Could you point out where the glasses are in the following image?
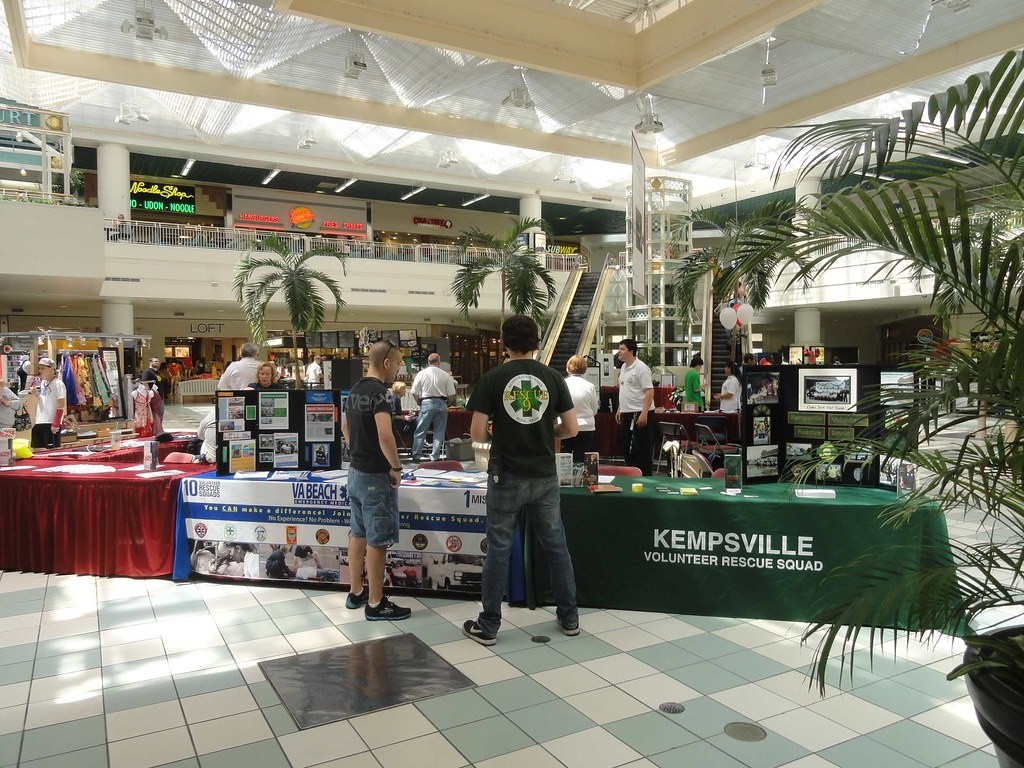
[382,338,396,369]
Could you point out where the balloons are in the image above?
[719,303,754,330]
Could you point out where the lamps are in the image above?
[552,164,576,184]
[297,127,317,149]
[761,38,776,86]
[436,145,458,169]
[115,101,149,124]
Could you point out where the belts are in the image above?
[420,396,446,402]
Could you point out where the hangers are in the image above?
[63,350,100,359]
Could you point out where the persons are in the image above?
[305,355,325,389]
[614,339,657,476]
[713,352,756,443]
[182,356,194,380]
[16,356,32,392]
[195,542,262,577]
[0,358,67,448]
[340,340,416,623]
[197,342,284,463]
[265,545,342,580]
[131,384,165,435]
[410,352,456,463]
[462,316,580,646]
[110,214,131,242]
[684,356,710,412]
[141,357,172,433]
[166,362,180,395]
[555,355,598,462]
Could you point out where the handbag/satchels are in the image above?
[12,404,32,431]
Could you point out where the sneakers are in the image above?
[365,594,411,621]
[556,617,581,636]
[462,619,497,645]
[345,585,369,609]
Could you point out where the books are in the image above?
[271,470,311,480]
[234,470,271,480]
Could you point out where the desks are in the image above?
[0,408,974,639]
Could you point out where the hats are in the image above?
[33,358,56,370]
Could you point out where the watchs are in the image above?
[393,463,403,472]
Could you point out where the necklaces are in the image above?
[43,375,55,387]
